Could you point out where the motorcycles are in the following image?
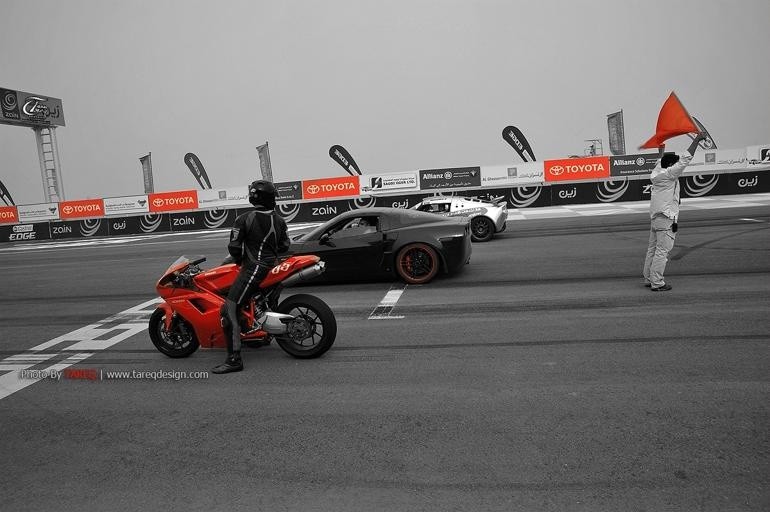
[147,251,338,360]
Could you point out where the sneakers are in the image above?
[211,358,244,374]
[651,283,672,291]
[645,283,651,287]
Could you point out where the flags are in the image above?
[639,134,666,150]
[655,92,699,145]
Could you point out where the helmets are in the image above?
[249,180,280,206]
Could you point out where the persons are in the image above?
[210,179,292,374]
[638,131,710,293]
[439,203,449,214]
[357,218,374,234]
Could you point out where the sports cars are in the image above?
[404,193,510,244]
[221,204,473,285]
